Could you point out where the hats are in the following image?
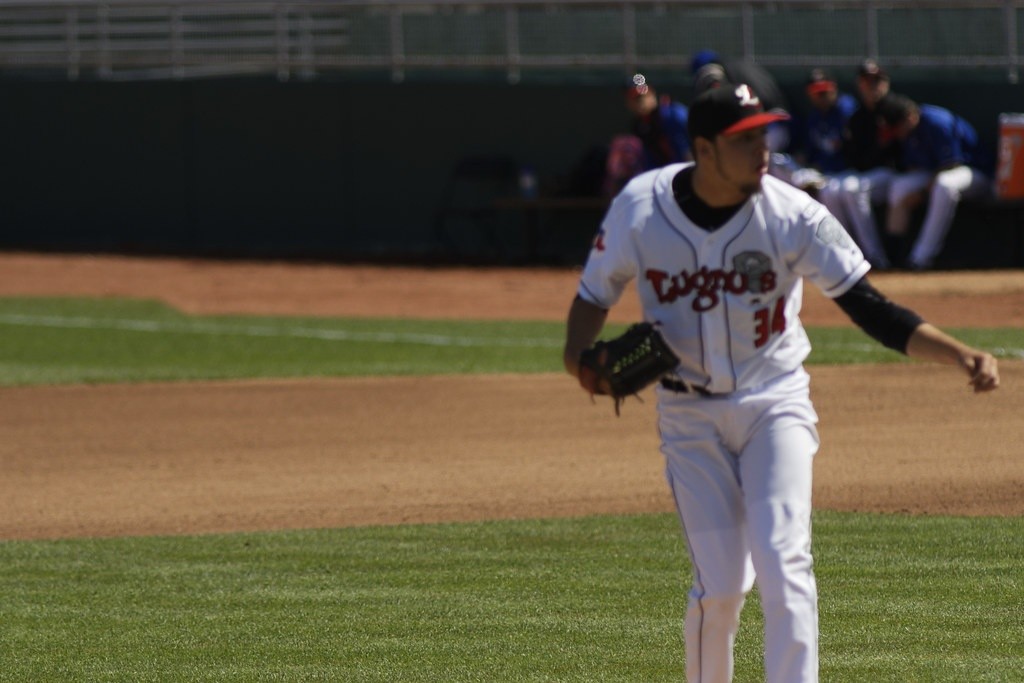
[687,84,791,140]
[622,70,657,104]
[852,57,891,80]
[690,52,727,91]
[806,68,837,95]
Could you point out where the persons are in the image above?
[562,82,998,683]
[606,52,985,268]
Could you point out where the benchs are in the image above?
[478,198,1024,264]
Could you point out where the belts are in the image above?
[658,378,711,398]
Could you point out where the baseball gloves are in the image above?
[578,321,681,399]
